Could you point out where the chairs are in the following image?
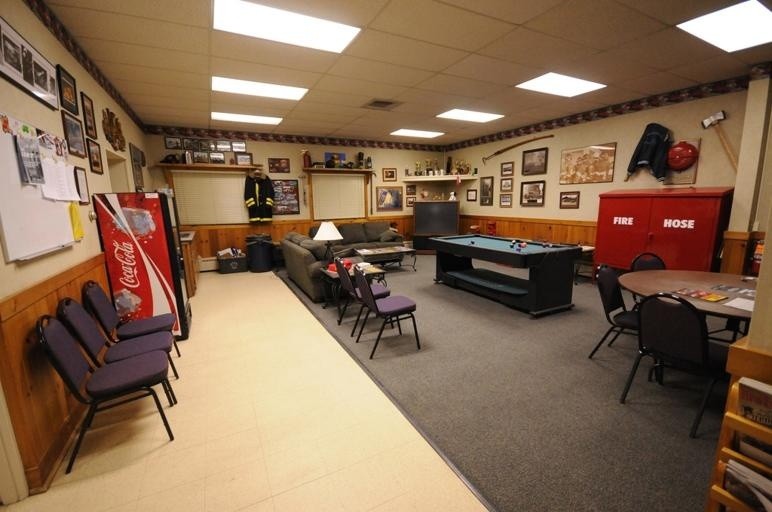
[36,313,174,474]
[588,263,641,360]
[620,293,730,441]
[334,259,393,337]
[56,298,179,404]
[353,265,421,359]
[82,280,181,377]
[630,253,666,312]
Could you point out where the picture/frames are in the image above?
[61,109,87,159]
[558,141,617,186]
[74,167,91,205]
[500,194,511,208]
[129,142,144,190]
[501,162,514,177]
[0,15,59,112]
[193,151,209,163]
[479,176,493,206]
[559,191,580,209]
[215,139,231,153]
[267,158,291,174]
[86,139,104,174]
[165,135,181,150]
[198,139,215,152]
[182,138,196,150]
[232,141,248,153]
[405,197,416,207]
[467,189,476,201]
[500,178,512,192]
[56,64,79,116]
[519,181,546,206]
[376,186,403,212]
[208,152,226,163]
[234,153,253,165]
[80,91,98,140]
[521,147,548,176]
[269,180,300,215]
[382,167,397,182]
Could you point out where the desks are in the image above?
[618,269,759,384]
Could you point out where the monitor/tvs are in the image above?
[412,201,459,236]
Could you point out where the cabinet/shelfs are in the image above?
[181,231,200,298]
[592,186,734,287]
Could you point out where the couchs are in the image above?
[310,222,404,250]
[280,232,372,303]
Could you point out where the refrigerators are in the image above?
[92,192,192,342]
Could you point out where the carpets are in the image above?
[272,254,772,512]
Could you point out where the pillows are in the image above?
[376,230,397,242]
[333,248,355,259]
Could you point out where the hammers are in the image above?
[700,111,738,173]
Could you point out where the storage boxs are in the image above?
[218,254,249,274]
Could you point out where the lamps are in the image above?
[313,221,343,270]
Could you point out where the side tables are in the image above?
[319,261,388,325]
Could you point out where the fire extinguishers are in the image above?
[750,238,764,274]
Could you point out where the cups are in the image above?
[429,171,433,176]
